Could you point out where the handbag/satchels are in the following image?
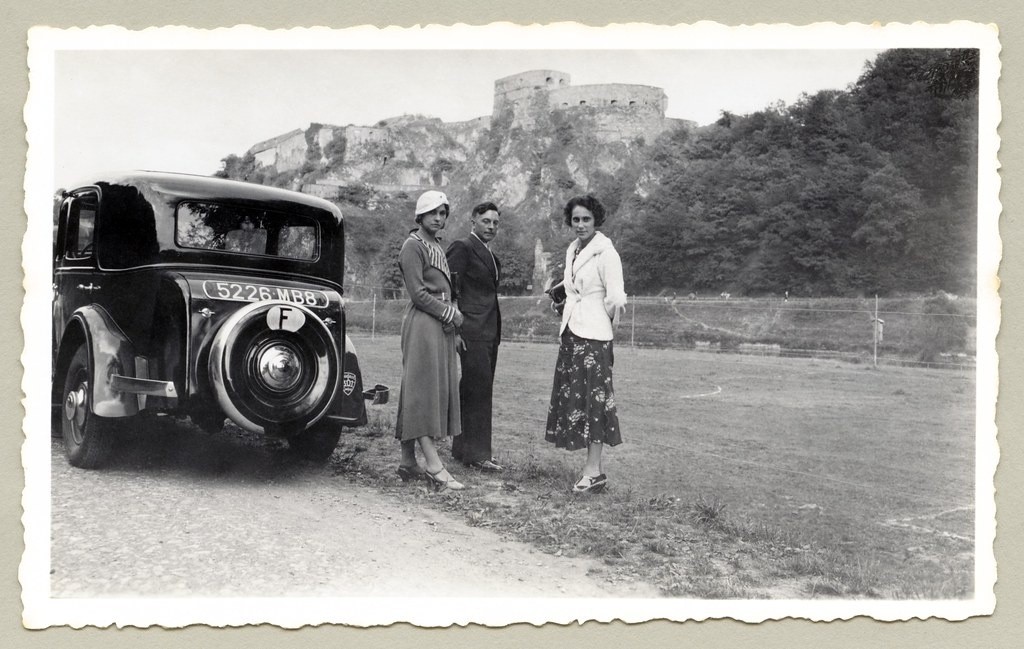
[543,279,566,315]
[450,271,462,302]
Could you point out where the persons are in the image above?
[446,202,503,473]
[545,197,625,494]
[395,190,464,489]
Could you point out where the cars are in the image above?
[53,170,390,469]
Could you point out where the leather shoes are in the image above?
[453,454,498,464]
[464,459,503,471]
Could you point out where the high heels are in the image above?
[426,466,464,490]
[572,472,608,496]
[398,463,427,482]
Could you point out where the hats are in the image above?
[414,189,450,215]
